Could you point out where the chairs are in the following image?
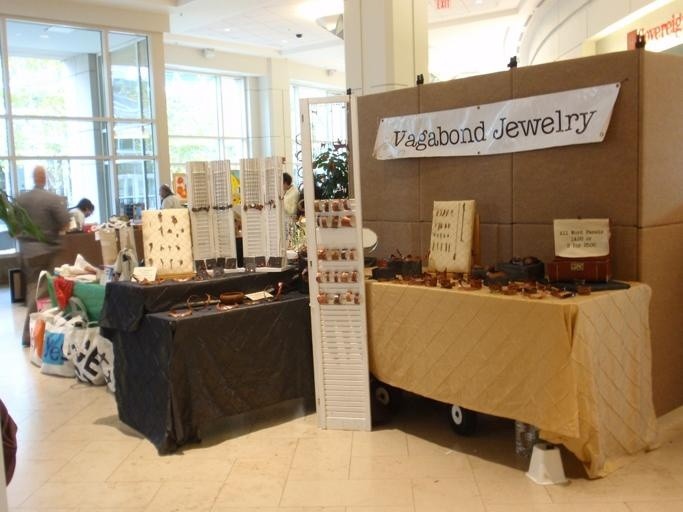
[61,230,144,267]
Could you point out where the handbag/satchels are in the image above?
[29,296,107,386]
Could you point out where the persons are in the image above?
[15,165,69,307]
[159,184,183,209]
[68,198,95,233]
[283,173,300,215]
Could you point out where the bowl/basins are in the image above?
[220,291,244,304]
